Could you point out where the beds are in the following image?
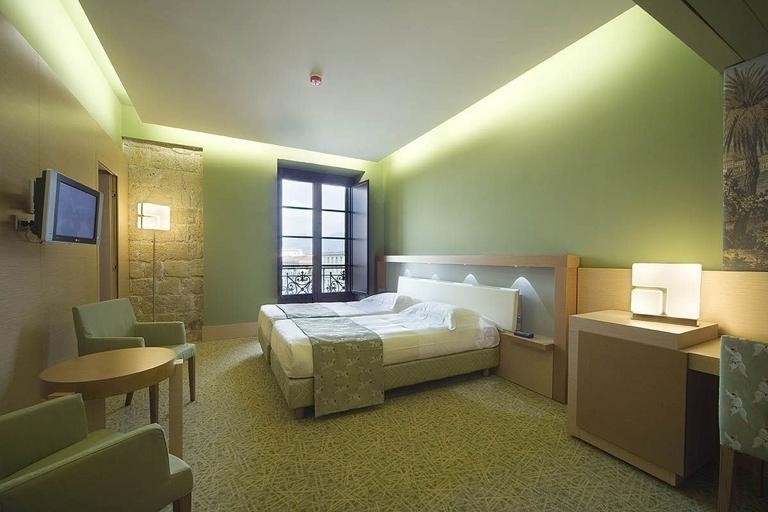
[267,315,502,420]
[255,301,419,370]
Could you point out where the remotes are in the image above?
[514,329,535,338]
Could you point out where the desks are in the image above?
[565,309,725,489]
[37,347,186,461]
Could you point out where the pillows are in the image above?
[363,291,419,310]
[406,299,481,330]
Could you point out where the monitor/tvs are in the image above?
[30,168,104,245]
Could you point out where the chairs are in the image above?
[0,388,194,512]
[710,331,768,512]
[69,295,197,425]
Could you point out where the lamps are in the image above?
[630,261,702,327]
[135,200,173,322]
